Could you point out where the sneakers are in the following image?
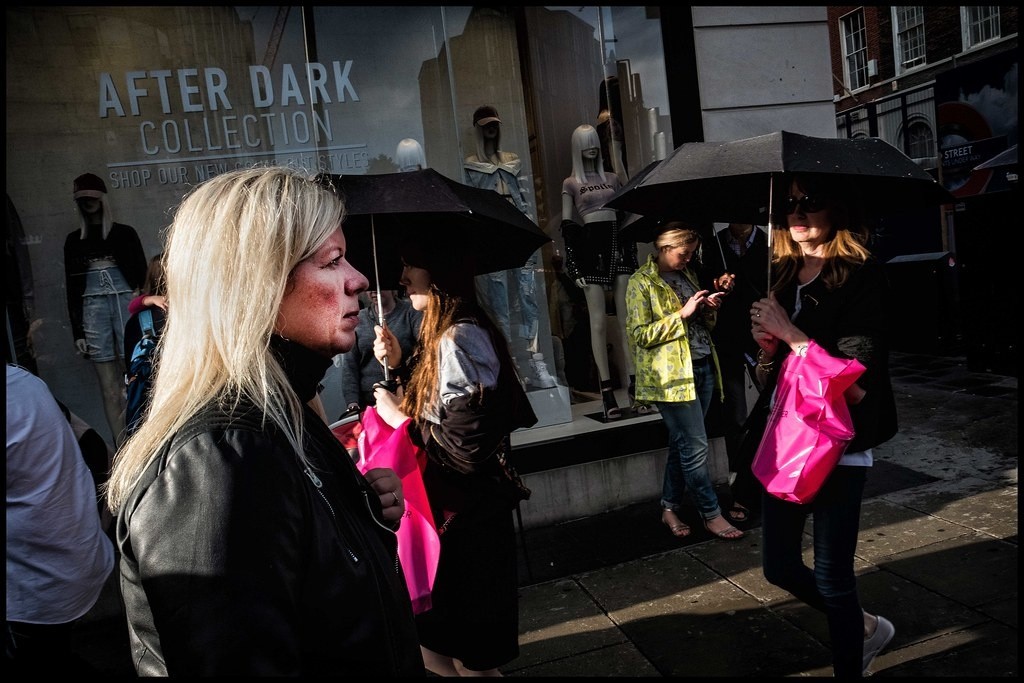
[861,616,895,675]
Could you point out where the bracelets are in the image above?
[759,363,773,372]
[756,348,775,366]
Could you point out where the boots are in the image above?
[511,357,526,393]
[529,353,558,389]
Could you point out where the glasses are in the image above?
[783,193,826,215]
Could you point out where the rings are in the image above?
[390,490,400,505]
[755,307,761,317]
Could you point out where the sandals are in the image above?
[728,497,750,522]
[703,512,746,540]
[662,507,692,538]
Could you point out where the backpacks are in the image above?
[125,312,160,437]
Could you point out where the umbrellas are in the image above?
[305,166,554,393]
[601,130,940,301]
[600,159,728,274]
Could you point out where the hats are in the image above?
[73,173,108,201]
[473,105,503,127]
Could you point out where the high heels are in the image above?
[602,386,622,419]
[627,381,652,414]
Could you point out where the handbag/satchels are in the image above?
[748,335,866,505]
[354,405,441,615]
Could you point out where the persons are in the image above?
[463,106,557,391]
[342,290,425,416]
[560,74,657,421]
[6,255,168,677]
[98,162,433,677]
[624,178,899,678]
[360,232,538,677]
[63,173,145,455]
[396,139,425,173]
